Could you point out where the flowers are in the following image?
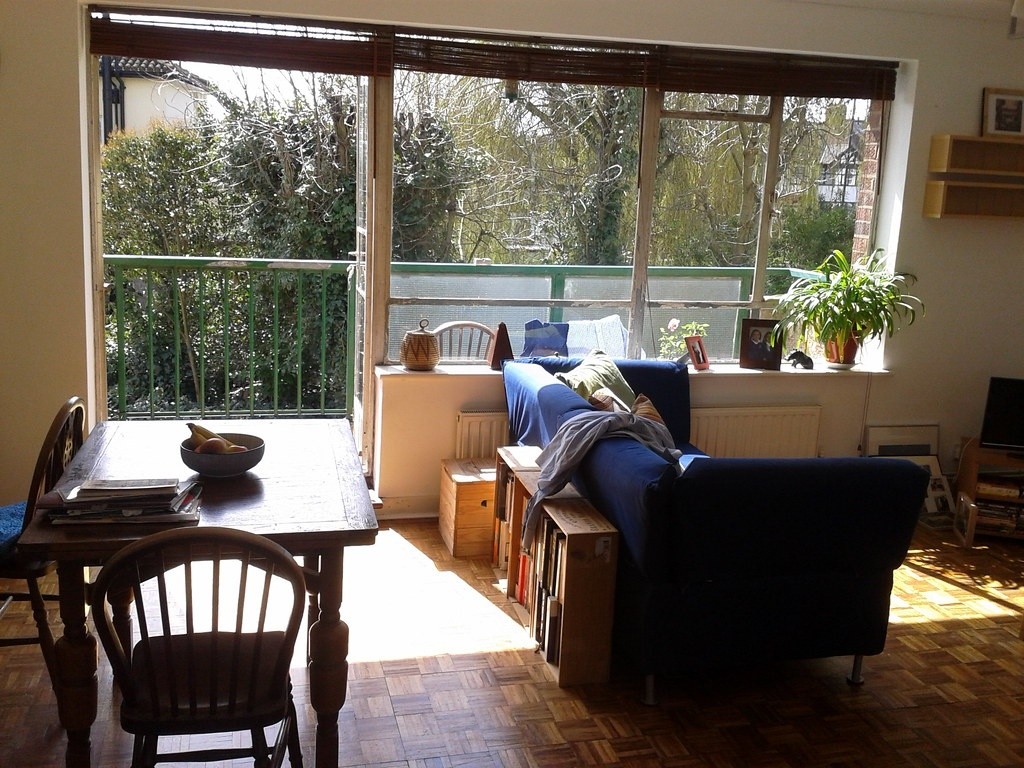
[658,318,710,363]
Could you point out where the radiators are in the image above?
[456,408,820,460]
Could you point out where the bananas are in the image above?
[187,423,235,447]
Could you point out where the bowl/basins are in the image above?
[179,433,266,476]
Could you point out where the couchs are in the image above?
[503,359,925,691]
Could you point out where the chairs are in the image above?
[91,525,307,767]
[1,399,91,726]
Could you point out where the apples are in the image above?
[195,438,249,452]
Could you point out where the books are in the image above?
[35,479,204,525]
[492,462,566,666]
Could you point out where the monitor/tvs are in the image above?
[979,376,1024,460]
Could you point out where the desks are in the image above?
[14,420,377,767]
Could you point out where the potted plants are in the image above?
[771,248,925,370]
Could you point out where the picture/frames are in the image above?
[683,336,710,370]
[741,319,784,370]
[982,87,1024,136]
[865,424,943,477]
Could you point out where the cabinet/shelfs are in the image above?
[492,444,619,685]
[439,458,496,557]
[925,133,1024,220]
[960,437,1023,539]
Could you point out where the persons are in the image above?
[692,345,701,364]
[749,329,775,358]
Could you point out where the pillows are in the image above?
[555,350,636,411]
